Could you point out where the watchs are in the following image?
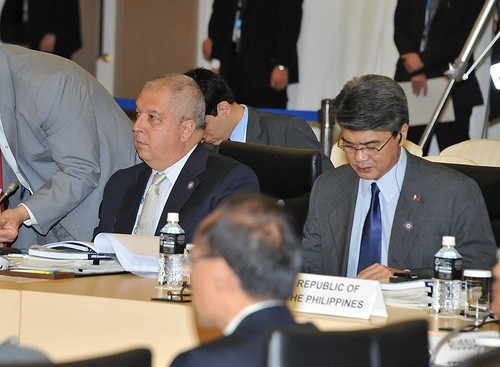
[278,64,286,71]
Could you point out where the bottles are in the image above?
[157,212,185,290]
[429,236,461,313]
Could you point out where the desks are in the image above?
[0,245,484,367]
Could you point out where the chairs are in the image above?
[217,138,500,278]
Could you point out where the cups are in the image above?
[461,269,494,319]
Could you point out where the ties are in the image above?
[429,0,439,22]
[356,183,382,275]
[135,172,167,237]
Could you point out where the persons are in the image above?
[171,194,323,367]
[202,0,303,110]
[450,262,500,367]
[0,41,142,251]
[296,74,495,283]
[0,0,84,61]
[394,0,486,157]
[189,68,336,177]
[90,74,261,258]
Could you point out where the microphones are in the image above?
[0,179,20,204]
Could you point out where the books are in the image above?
[382,279,466,309]
[2,233,162,273]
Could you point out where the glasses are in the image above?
[336,130,396,157]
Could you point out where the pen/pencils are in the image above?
[10,270,56,274]
